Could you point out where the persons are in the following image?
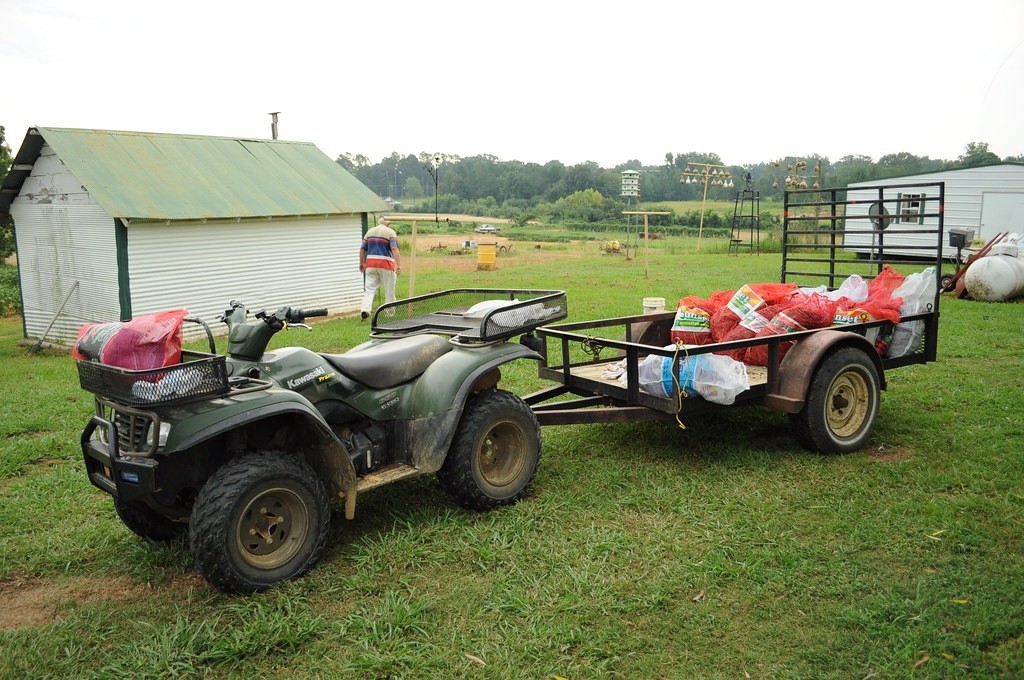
[358,216,403,321]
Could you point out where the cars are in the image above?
[475,224,497,233]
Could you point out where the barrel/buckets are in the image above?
[478,244,496,271]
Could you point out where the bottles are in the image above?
[132,380,162,400]
[158,366,206,395]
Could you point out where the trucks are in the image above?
[74,182,946,595]
